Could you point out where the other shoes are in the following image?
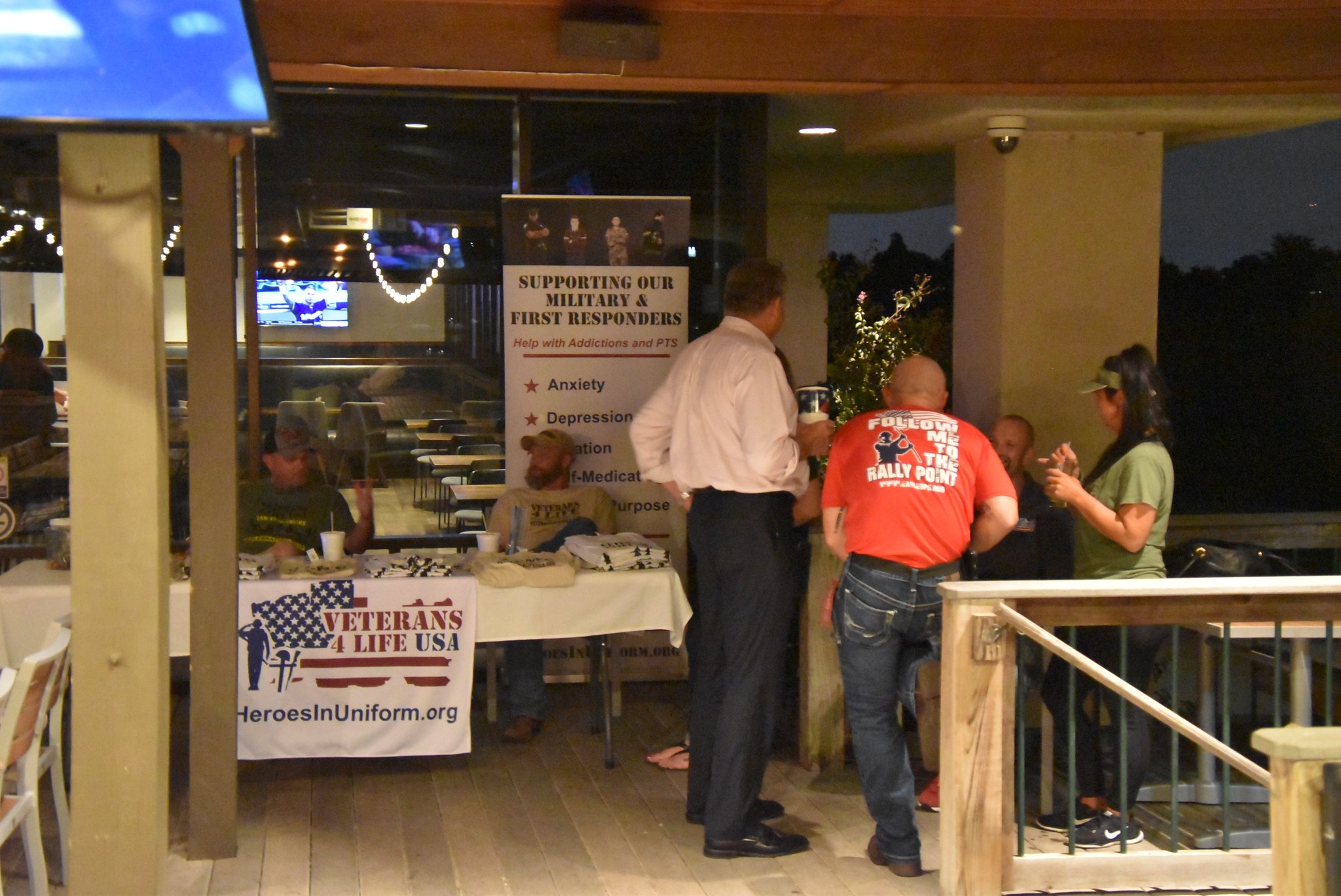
[648,740,690,771]
[868,834,922,877]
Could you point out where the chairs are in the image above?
[333,402,390,489]
[411,401,505,554]
[274,401,330,483]
[0,626,72,896]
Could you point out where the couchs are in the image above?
[47,364,457,477]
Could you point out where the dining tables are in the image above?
[48,401,68,443]
[0,553,674,771]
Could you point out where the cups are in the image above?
[796,386,829,424]
[1048,459,1074,508]
[320,531,346,562]
[475,532,502,553]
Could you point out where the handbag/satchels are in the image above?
[1173,537,1307,575]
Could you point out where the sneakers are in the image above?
[918,772,940,812]
[1064,809,1144,847]
[1037,808,1100,831]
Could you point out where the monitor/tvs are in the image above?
[256,274,348,326]
[0,0,278,139]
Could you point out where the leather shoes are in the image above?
[703,826,809,858]
[499,716,539,743]
[686,799,784,823]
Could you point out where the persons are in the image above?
[606,216,630,266]
[916,415,1079,812]
[486,429,617,741]
[561,215,587,266]
[239,416,375,567]
[0,328,58,450]
[628,258,836,859]
[520,208,551,265]
[1037,344,1174,848]
[640,210,667,266]
[820,356,1019,878]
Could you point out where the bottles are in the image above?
[43,518,72,571]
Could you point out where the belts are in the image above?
[850,552,961,579]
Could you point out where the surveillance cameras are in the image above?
[986,114,1028,154]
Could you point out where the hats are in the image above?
[1076,368,1157,397]
[521,429,575,464]
[0,328,43,357]
[261,430,318,460]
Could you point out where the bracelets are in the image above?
[674,491,694,509]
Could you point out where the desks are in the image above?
[429,454,506,531]
[416,433,505,507]
[1137,620,1341,849]
[404,419,505,477]
[261,408,341,414]
[450,485,505,553]
